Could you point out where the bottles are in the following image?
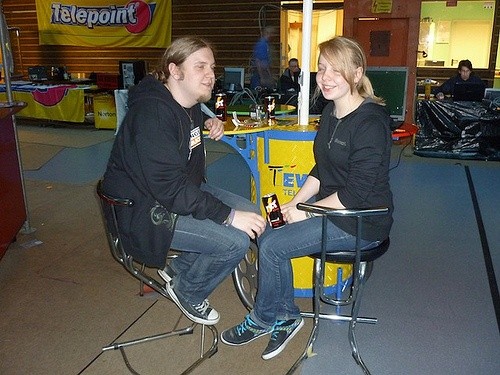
[425,80,430,100]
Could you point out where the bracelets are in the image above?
[222,208,236,228]
[305,210,315,218]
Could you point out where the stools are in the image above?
[96,178,219,375]
[283,202,391,375]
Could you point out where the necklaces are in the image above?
[328,94,360,140]
[165,80,193,124]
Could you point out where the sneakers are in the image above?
[262,317,304,360]
[165,279,221,325]
[220,314,275,346]
[158,262,210,306]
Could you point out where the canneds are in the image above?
[214,93,227,123]
[264,96,276,118]
[262,193,286,230]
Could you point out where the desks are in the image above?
[209,97,297,115]
[202,116,278,203]
[0,81,99,127]
[388,122,419,171]
[414,98,500,161]
[92,93,117,130]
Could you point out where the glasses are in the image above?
[290,65,299,68]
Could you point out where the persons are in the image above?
[276,58,301,115]
[249,24,273,93]
[434,60,489,102]
[98,36,268,326]
[220,36,395,361]
